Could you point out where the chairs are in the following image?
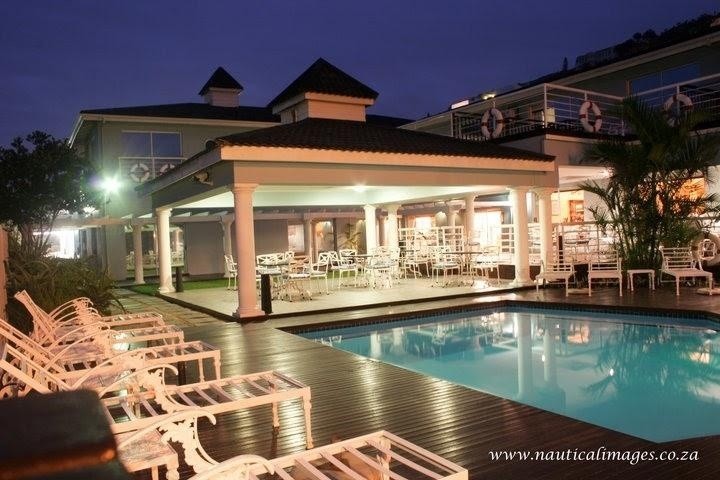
[0,282,469,480]
[124,244,713,303]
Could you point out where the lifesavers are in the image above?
[692,231,720,267]
[662,94,693,128]
[579,100,602,132]
[481,108,504,140]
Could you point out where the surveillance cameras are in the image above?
[193,171,209,184]
